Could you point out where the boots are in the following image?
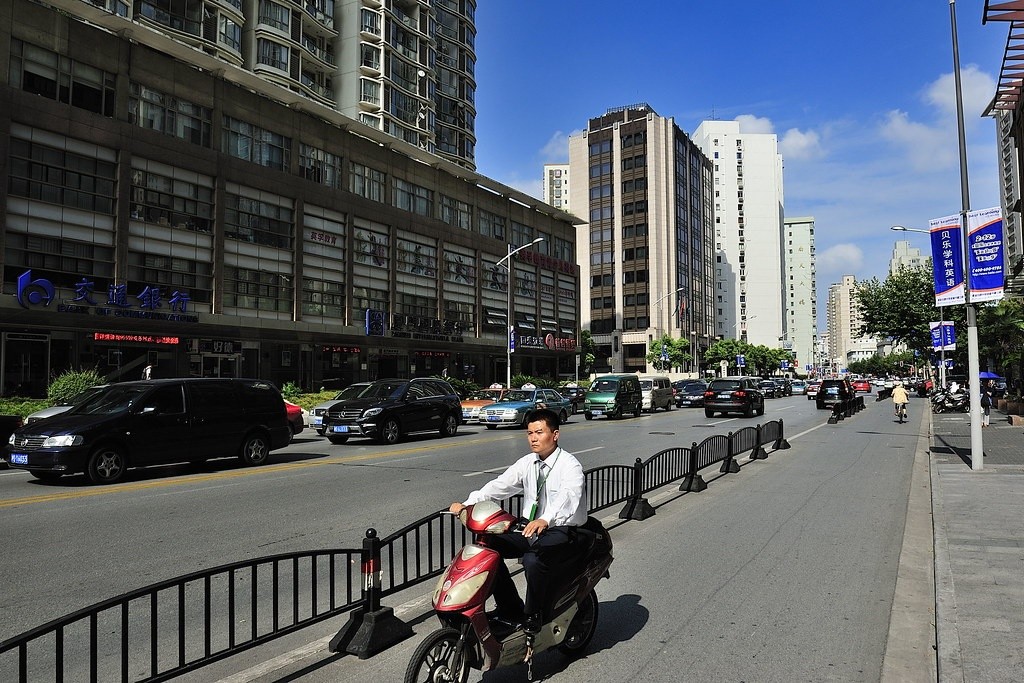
[903,409,907,419]
[895,409,898,416]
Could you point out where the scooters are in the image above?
[930,382,971,414]
[403,499,614,683]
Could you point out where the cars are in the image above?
[852,380,872,394]
[847,373,923,389]
[995,376,1006,392]
[671,378,711,409]
[750,376,822,401]
[946,375,968,384]
[283,401,305,441]
[307,380,377,437]
[559,382,590,414]
[460,382,517,426]
[478,383,573,430]
[22,384,110,428]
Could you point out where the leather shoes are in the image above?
[486,608,510,622]
[522,612,541,633]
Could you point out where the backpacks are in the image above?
[141,367,151,380]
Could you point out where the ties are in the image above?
[527,462,547,547]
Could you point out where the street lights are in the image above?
[495,237,544,391]
[732,314,758,376]
[778,327,800,379]
[652,287,685,373]
[890,225,947,391]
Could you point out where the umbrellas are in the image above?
[979,372,999,378]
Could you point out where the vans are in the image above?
[583,373,643,420]
[638,376,675,413]
[3,377,292,485]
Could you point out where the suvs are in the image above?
[703,376,764,418]
[321,376,464,444]
[816,379,854,409]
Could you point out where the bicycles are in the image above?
[896,402,906,424]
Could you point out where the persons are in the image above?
[891,382,909,419]
[955,385,965,394]
[920,380,926,398]
[142,363,154,380]
[449,410,587,637]
[980,380,992,428]
